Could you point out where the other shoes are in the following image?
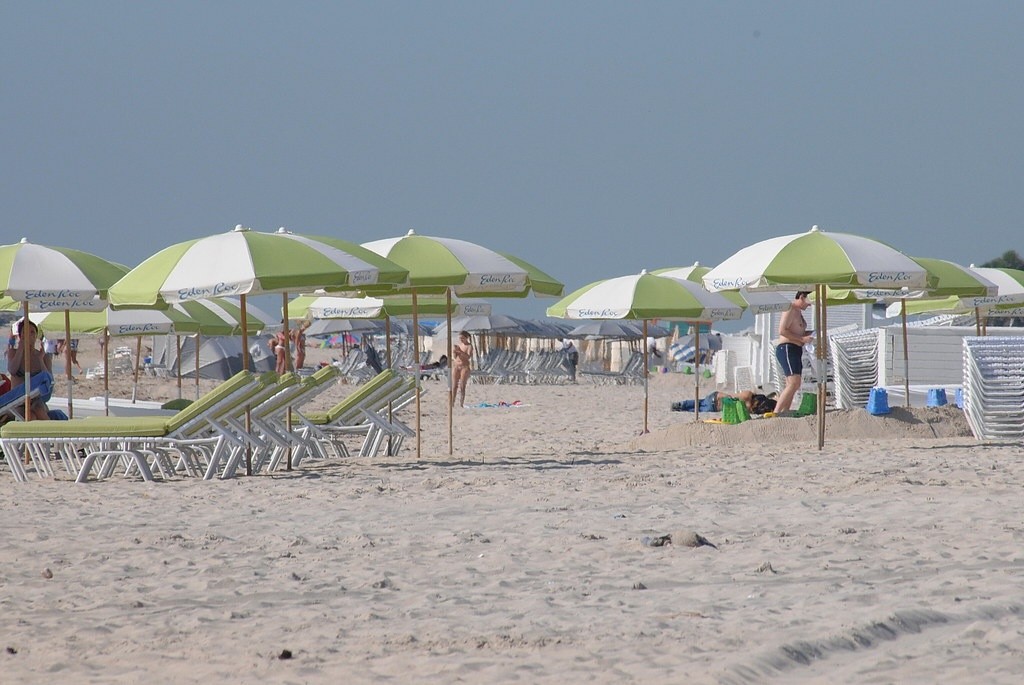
[671,401,679,412]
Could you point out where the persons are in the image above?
[399,355,447,371]
[772,292,817,418]
[318,344,359,369]
[449,330,473,410]
[555,335,579,385]
[673,391,775,414]
[7,319,53,421]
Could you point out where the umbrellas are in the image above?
[107,224,411,476]
[702,224,940,450]
[0,221,1023,476]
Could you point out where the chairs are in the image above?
[713,301,1024,440]
[467,347,646,386]
[0,347,450,483]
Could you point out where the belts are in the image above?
[711,391,717,412]
